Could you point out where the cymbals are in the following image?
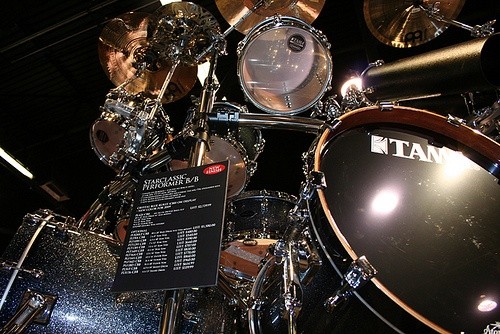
[95,11,199,105]
[363,0,465,49]
[216,0,326,42]
[147,2,221,67]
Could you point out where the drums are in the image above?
[246,99,500,334]
[116,179,138,245]
[236,12,335,116]
[89,89,170,174]
[164,96,266,202]
[217,189,300,283]
